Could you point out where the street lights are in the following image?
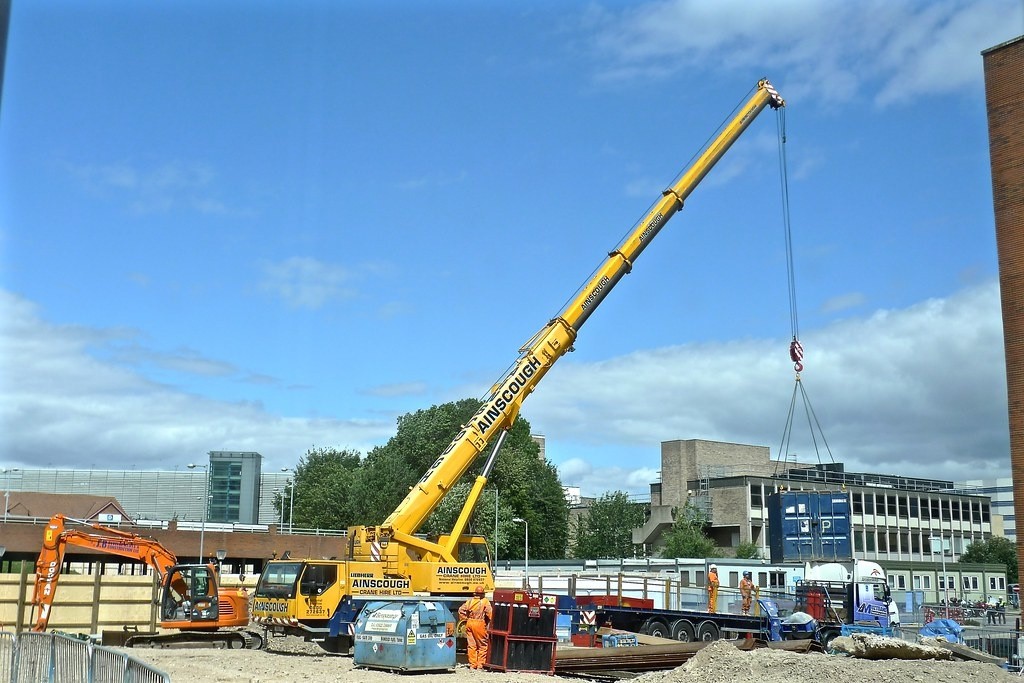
[2,469,20,515]
[186,463,208,565]
[928,537,950,620]
[513,517,529,591]
[281,467,294,535]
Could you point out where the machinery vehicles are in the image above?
[252,79,784,652]
[29,514,262,650]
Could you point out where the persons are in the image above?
[987,596,1006,625]
[708,564,719,614]
[458,585,493,672]
[740,570,756,616]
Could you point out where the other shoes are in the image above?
[469,668,487,673]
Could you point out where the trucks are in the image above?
[556,559,902,655]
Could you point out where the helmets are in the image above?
[998,596,1003,599]
[710,563,717,569]
[743,570,750,576]
[474,586,488,593]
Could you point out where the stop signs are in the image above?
[1013,585,1021,593]
